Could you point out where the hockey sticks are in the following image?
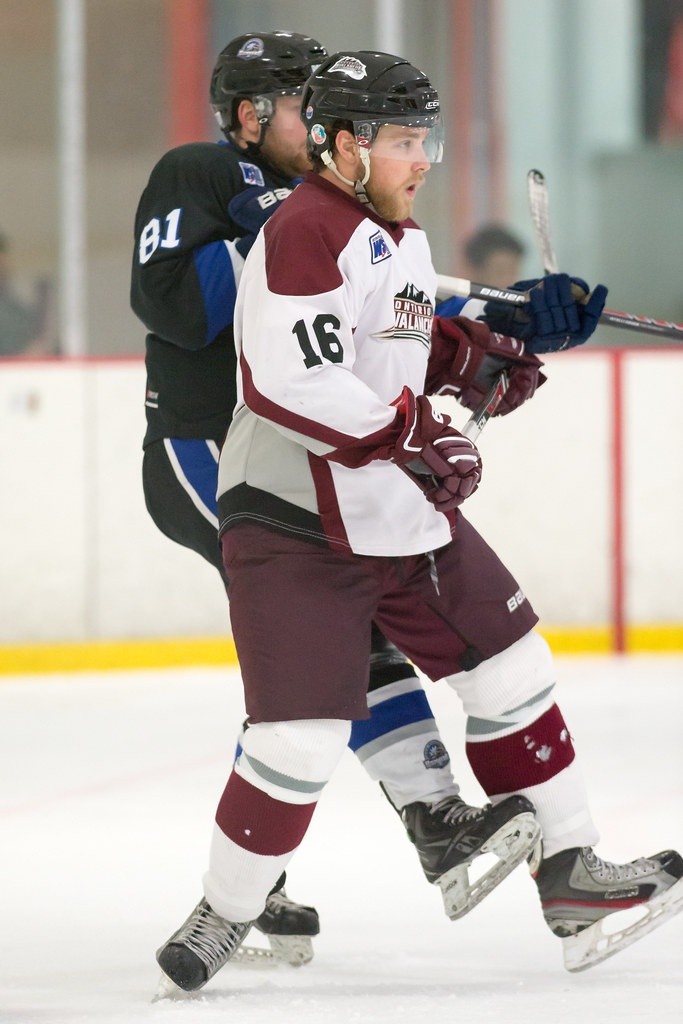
[434,273,683,344]
[423,168,560,490]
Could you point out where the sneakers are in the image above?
[229,869,320,971]
[526,839,683,973]
[378,780,542,921]
[150,896,255,1005]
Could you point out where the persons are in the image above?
[463,226,527,289]
[130,31,610,969]
[1,231,59,357]
[149,50,683,1002]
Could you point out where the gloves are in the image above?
[228,186,294,255]
[388,385,482,512]
[475,273,608,354]
[423,316,547,417]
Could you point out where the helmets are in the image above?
[209,30,329,134]
[299,51,446,165]
